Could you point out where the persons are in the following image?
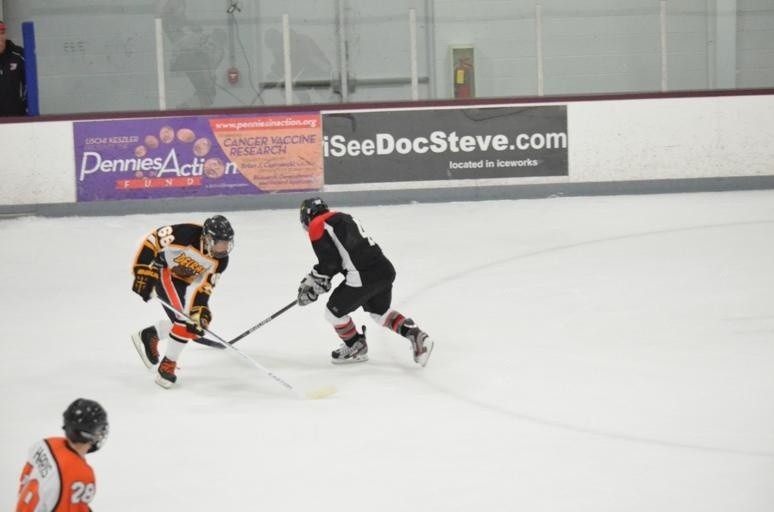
[0,21,27,117]
[132,215,233,388]
[297,199,431,365]
[15,399,107,511]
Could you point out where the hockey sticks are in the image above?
[192,300,298,349]
[150,292,337,398]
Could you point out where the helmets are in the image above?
[62,396,110,452]
[199,214,236,260]
[300,196,330,231]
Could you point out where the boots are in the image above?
[157,355,178,384]
[402,316,431,364]
[139,325,161,365]
[330,324,369,359]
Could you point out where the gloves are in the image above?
[296,269,332,307]
[131,275,157,303]
[186,290,212,336]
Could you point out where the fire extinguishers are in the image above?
[454,57,473,100]
